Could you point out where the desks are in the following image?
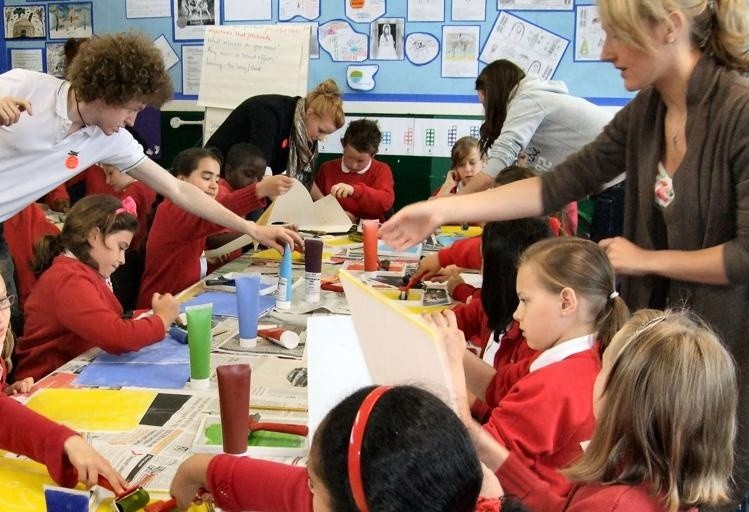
[1,223,483,511]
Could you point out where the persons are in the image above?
[1,1,749,512]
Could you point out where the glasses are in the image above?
[0,293,17,310]
